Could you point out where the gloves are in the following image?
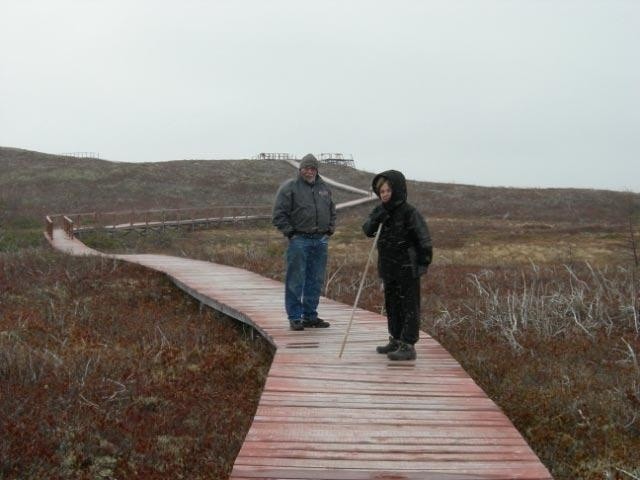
[374,208,391,224]
[417,248,433,267]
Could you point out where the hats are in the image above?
[299,154,319,173]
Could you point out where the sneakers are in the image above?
[377,335,402,354]
[290,319,304,331]
[302,317,330,328]
[388,341,417,360]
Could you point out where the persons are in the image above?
[271,153,338,331]
[361,171,433,361]
